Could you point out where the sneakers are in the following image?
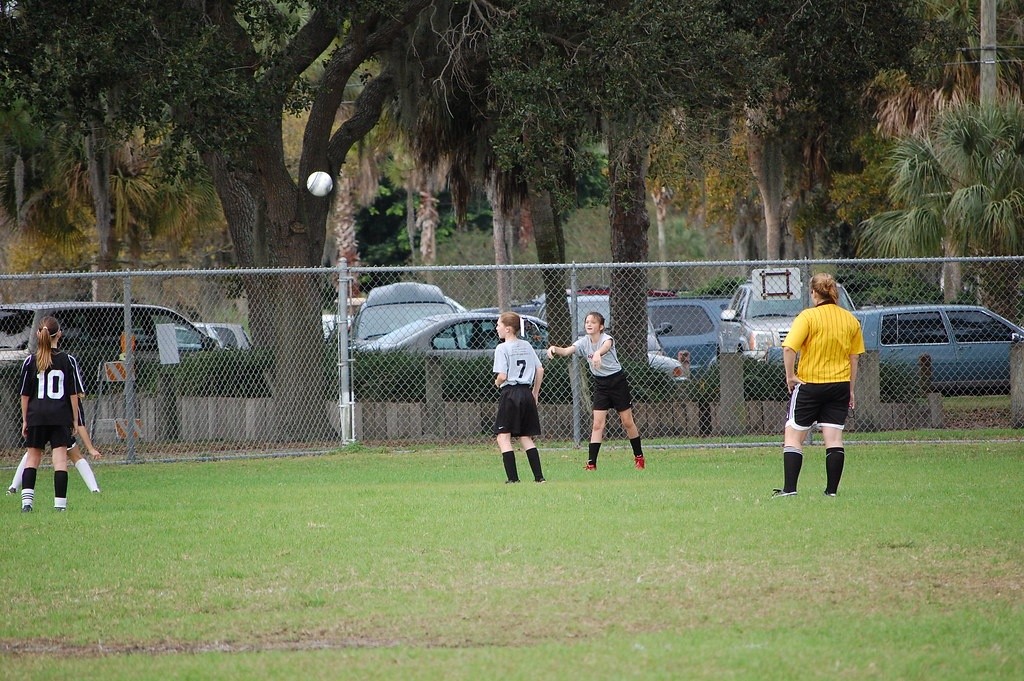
[582,460,596,471]
[634,455,645,470]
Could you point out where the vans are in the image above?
[352,283,469,346]
[0,302,220,360]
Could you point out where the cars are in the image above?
[358,313,585,360]
[322,297,365,340]
[192,323,252,350]
[851,305,1024,402]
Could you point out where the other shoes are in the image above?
[822,491,836,498]
[505,479,520,484]
[6,487,16,495]
[21,505,31,513]
[533,479,546,483]
[54,506,66,513]
[770,488,798,499]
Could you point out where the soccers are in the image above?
[306,170,334,198]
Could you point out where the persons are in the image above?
[769,273,865,499]
[548,312,645,470]
[493,310,546,484]
[6,317,103,511]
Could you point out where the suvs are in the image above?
[721,278,859,367]
[474,285,731,366]
